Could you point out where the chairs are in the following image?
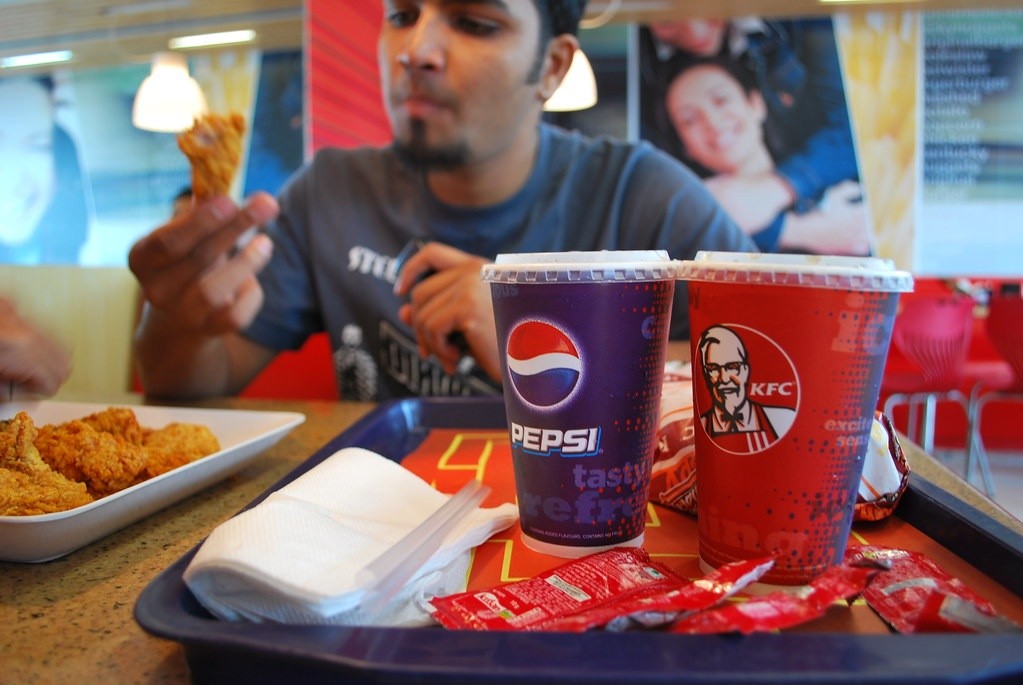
[888,291,1023,499]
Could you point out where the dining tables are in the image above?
[0,401,1023,685]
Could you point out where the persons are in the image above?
[638,17,872,257]
[126,186,193,397]
[126,1,762,404]
[0,75,98,267]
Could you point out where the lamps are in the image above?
[542,48,599,112]
[132,51,210,133]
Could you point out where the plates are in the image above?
[0,398,306,563]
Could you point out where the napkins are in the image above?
[181,447,520,625]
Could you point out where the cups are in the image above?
[677,250,914,596]
[482,249,672,559]
